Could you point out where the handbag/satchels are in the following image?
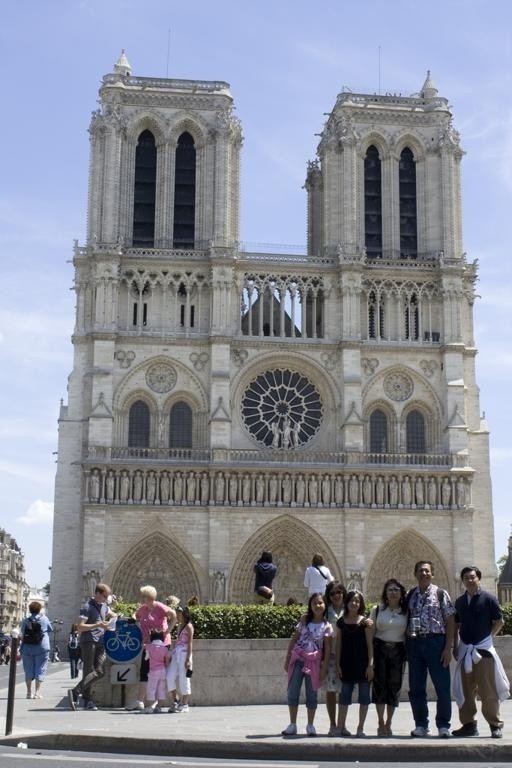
[255,584,274,600]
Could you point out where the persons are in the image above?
[281,554,374,737]
[0,584,198,715]
[452,566,510,738]
[371,577,409,737]
[406,561,458,737]
[253,550,277,606]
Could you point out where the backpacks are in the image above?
[68,633,80,650]
[23,617,45,645]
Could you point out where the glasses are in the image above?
[329,590,344,596]
[387,587,401,593]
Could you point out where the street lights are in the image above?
[52,615,66,663]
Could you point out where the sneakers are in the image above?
[451,721,479,737]
[411,726,429,738]
[438,727,450,738]
[281,724,297,735]
[377,727,392,737]
[490,722,503,738]
[327,724,351,737]
[357,728,367,738]
[305,725,317,736]
[26,693,44,700]
[67,689,189,714]
